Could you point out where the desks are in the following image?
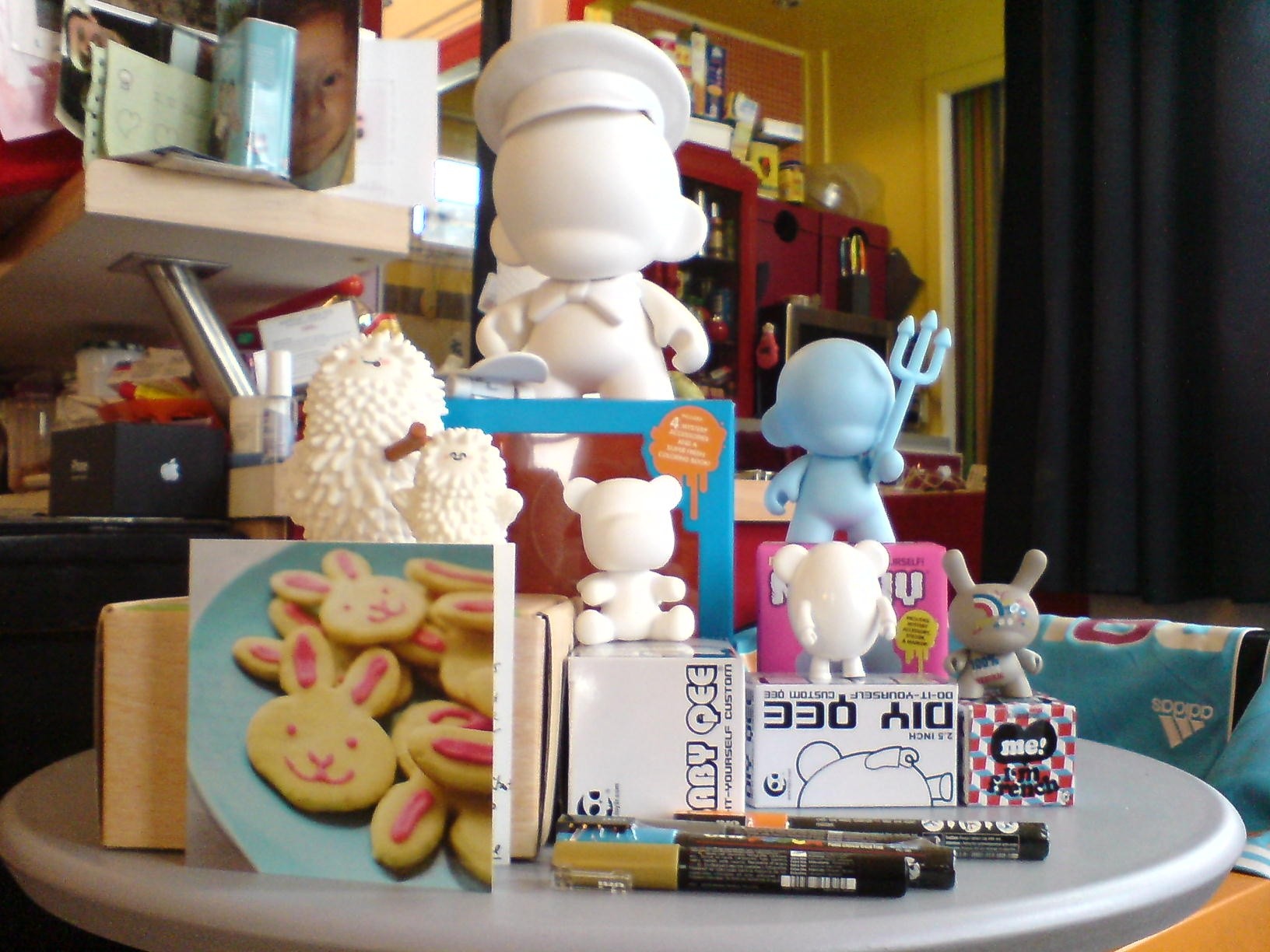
[0,715,1242,952]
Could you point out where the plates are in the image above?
[0,741,1247,952]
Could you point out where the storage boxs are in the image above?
[50,399,1079,896]
[214,19,298,176]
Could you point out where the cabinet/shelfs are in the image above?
[0,0,405,626]
[646,140,893,435]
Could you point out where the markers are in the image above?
[552,840,909,899]
[672,810,1050,863]
[554,814,957,891]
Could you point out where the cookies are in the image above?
[231,548,493,887]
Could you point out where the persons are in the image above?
[266,0,360,193]
[64,8,171,71]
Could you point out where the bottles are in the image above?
[705,294,729,341]
[708,202,723,257]
